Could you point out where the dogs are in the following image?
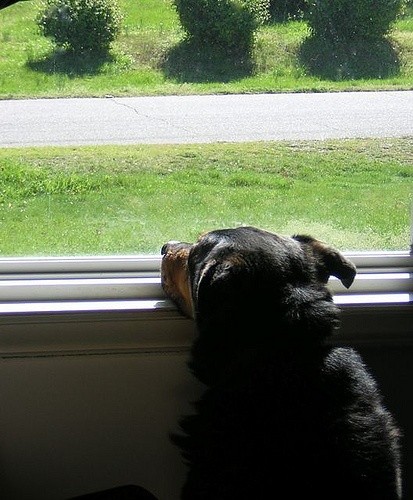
[159,224,403,500]
